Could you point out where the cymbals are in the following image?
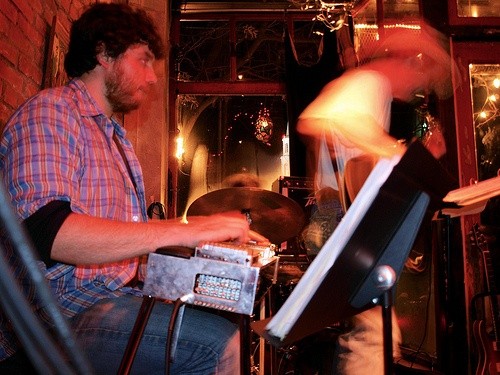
[187,188,306,241]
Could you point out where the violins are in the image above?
[469,224,500,375]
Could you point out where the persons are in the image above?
[0,1,262,374]
